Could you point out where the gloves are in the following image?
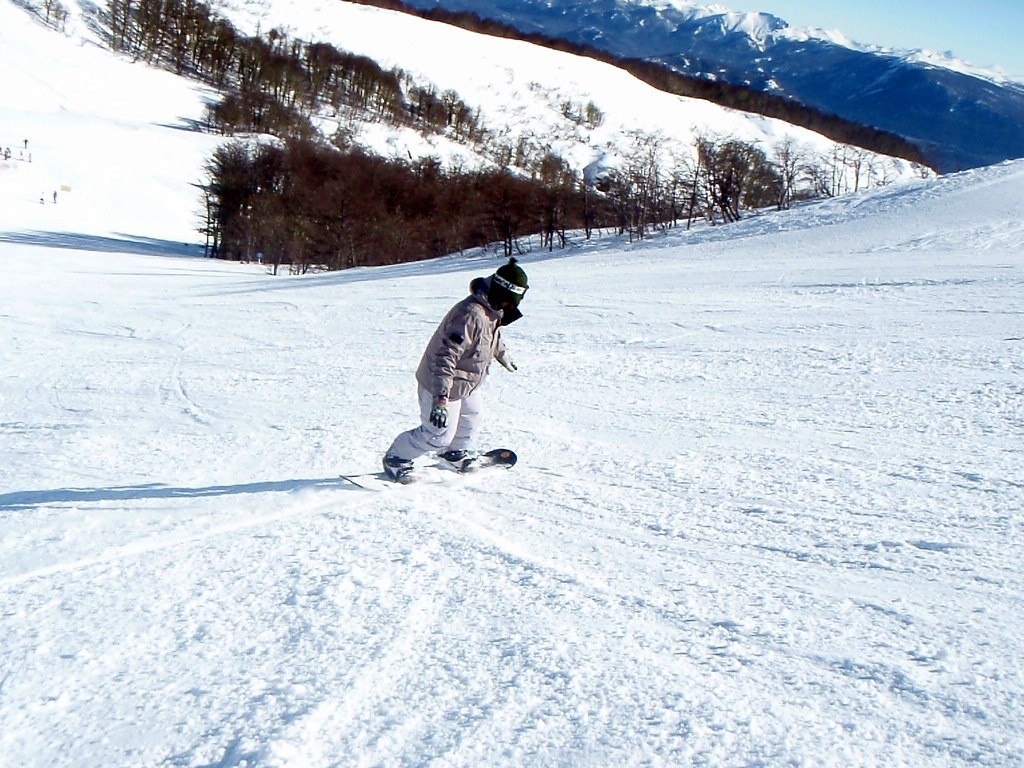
[495,350,518,372]
[429,394,449,429]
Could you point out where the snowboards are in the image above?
[339,448,518,491]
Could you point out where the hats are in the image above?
[488,258,529,307]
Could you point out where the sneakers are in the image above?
[436,448,481,474]
[382,455,413,485]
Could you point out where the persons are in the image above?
[40,191,57,204]
[0,139,31,161]
[382,258,528,484]
